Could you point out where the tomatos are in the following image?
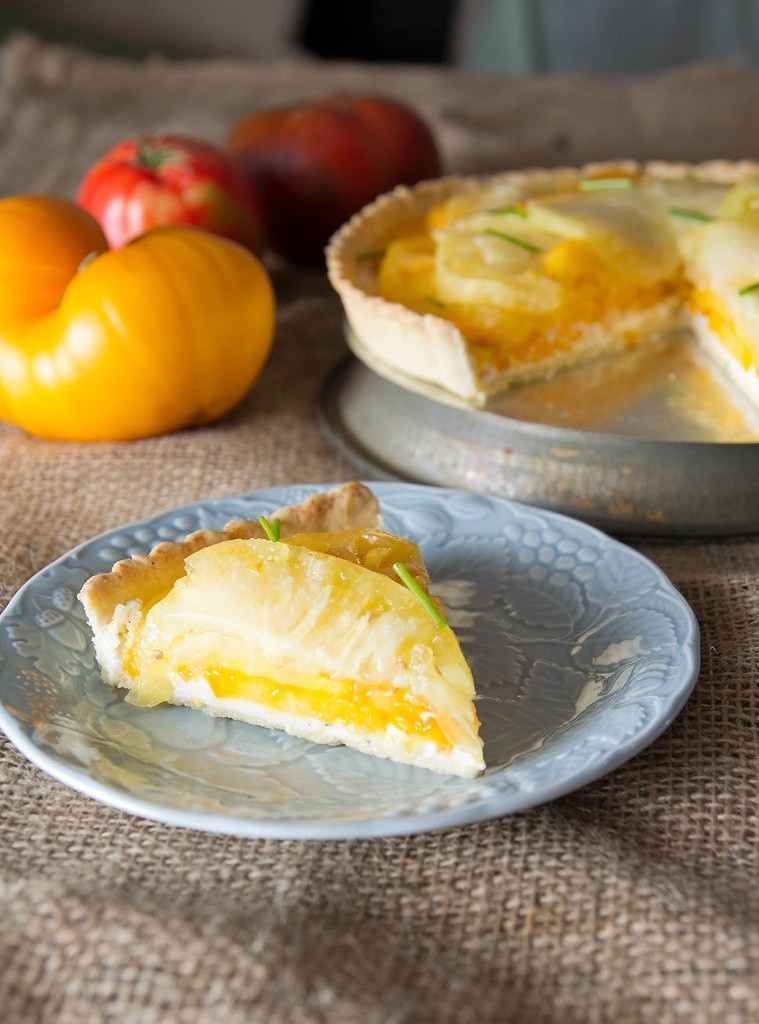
[1,92,442,440]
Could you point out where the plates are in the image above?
[0,479,702,842]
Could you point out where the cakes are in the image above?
[325,156,759,439]
[81,482,485,782]
